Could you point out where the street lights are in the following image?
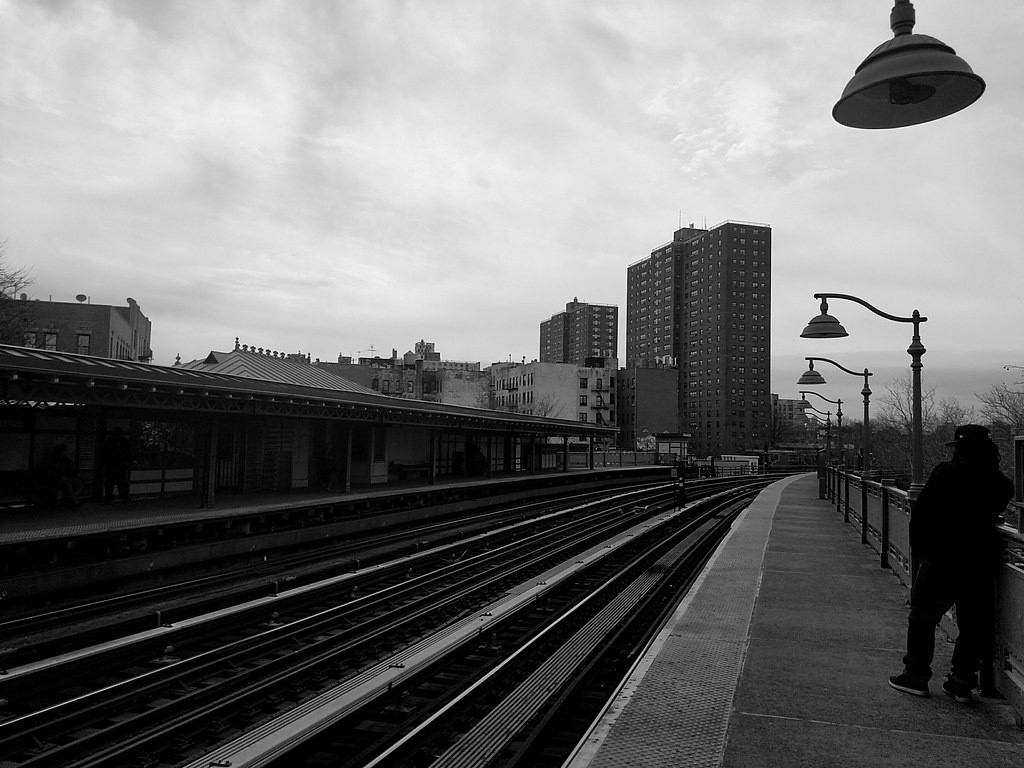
[800,294,927,499]
[795,404,832,464]
[797,357,874,479]
[794,391,843,470]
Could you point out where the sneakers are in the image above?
[942,680,975,703]
[889,672,929,696]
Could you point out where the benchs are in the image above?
[0,469,44,514]
[390,460,432,482]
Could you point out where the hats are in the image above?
[943,424,993,445]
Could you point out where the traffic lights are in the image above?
[678,476,684,488]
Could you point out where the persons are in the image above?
[99,427,130,504]
[889,425,1015,704]
